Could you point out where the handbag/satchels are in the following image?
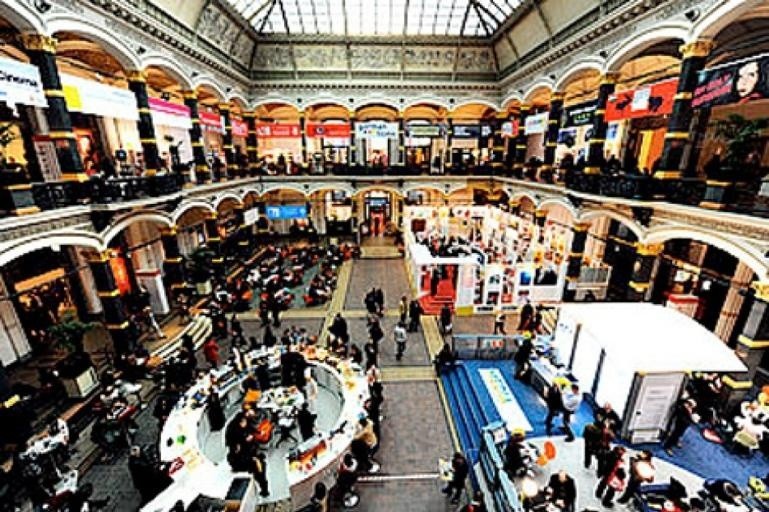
[608,473,625,492]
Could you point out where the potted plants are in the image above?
[41,317,102,400]
[187,245,220,296]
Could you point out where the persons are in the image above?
[726,59,768,103]
[0,134,768,512]
